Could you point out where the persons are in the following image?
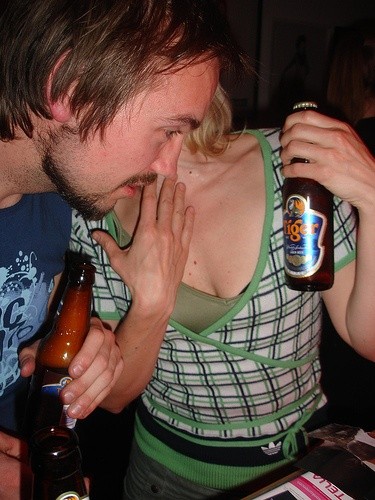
[0,0,220,500]
[61,81,375,500]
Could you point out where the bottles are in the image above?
[18,260,96,500]
[281,102,334,291]
